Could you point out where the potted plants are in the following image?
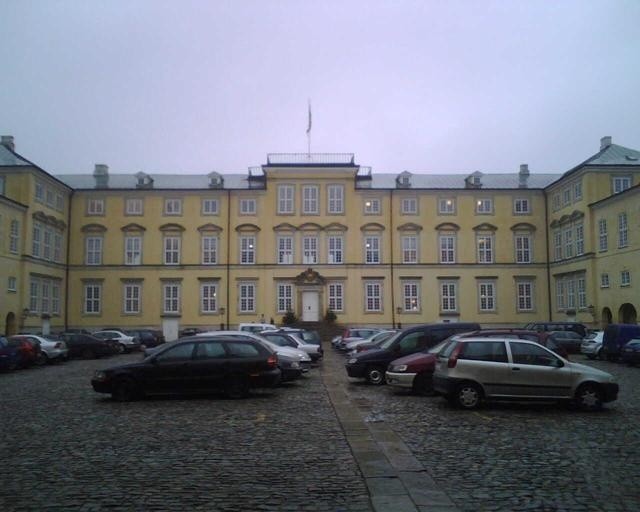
[325,311,337,323]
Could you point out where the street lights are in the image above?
[218,306,226,330]
[395,306,404,330]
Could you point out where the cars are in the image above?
[0,335,42,374]
[197,322,324,383]
[331,329,394,359]
[433,338,620,412]
[622,339,640,368]
[524,320,592,355]
[9,334,68,367]
[90,334,284,401]
[178,327,203,339]
[39,327,164,361]
[385,329,571,397]
[580,331,608,361]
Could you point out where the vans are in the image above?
[602,323,640,364]
[345,321,481,386]
